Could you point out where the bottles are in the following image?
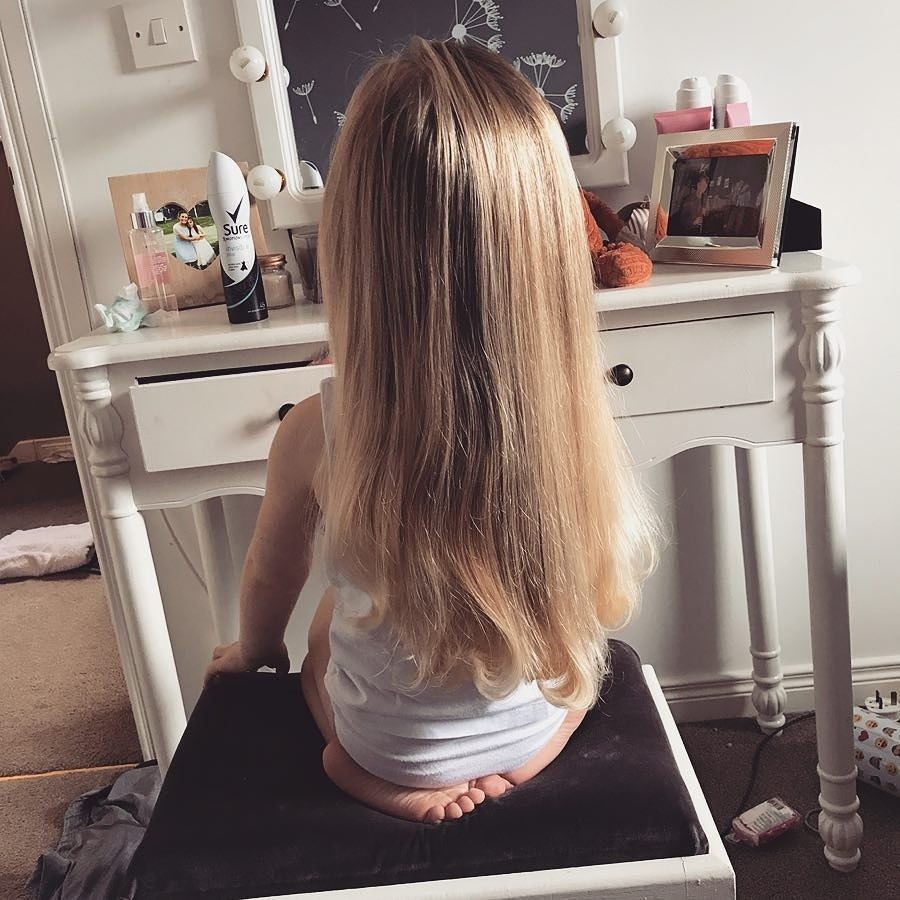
[257,254,295,310]
[207,151,268,325]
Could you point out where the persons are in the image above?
[172,210,217,269]
[676,173,710,236]
[203,38,664,825]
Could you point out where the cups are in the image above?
[292,230,323,304]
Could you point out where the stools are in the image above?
[129,638,737,900]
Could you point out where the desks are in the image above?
[46,248,864,873]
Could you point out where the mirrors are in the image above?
[232,0,630,231]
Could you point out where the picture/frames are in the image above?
[644,122,800,270]
[110,162,269,313]
